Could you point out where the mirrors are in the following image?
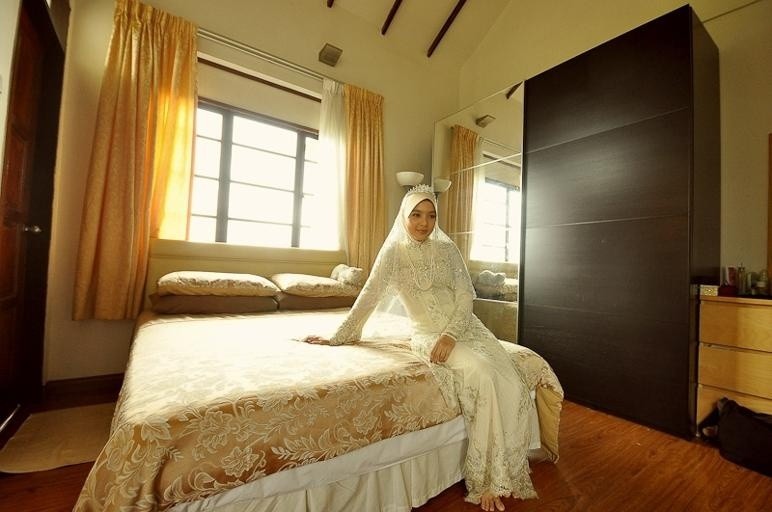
[431,82,522,303]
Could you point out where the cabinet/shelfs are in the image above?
[696,296,772,440]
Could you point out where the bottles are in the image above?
[737,262,768,295]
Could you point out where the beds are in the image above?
[69,262,563,512]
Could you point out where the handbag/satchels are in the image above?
[716,400,772,478]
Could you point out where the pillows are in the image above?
[273,273,359,296]
[148,293,277,313]
[470,270,518,302]
[276,292,354,309]
[330,264,362,284]
[156,270,280,297]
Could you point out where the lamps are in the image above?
[433,178,452,203]
[396,171,425,192]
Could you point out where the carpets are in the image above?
[0,398,115,476]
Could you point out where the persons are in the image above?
[303,183,541,512]
